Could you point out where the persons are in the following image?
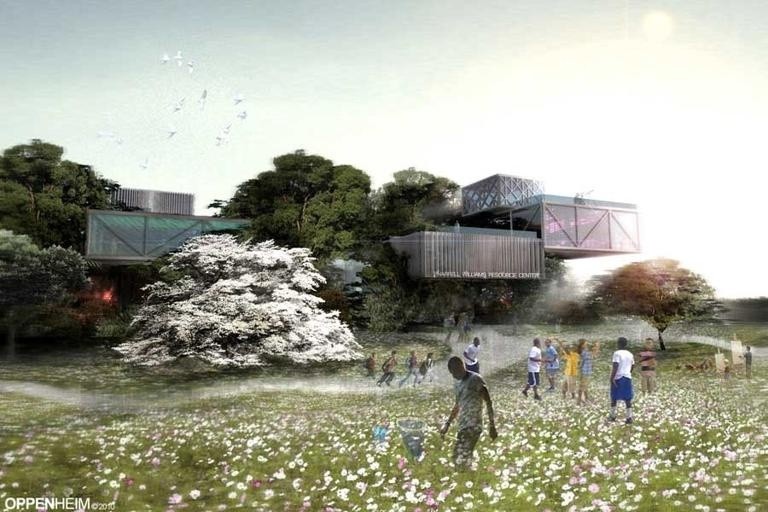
[399,349,419,388]
[439,355,497,469]
[463,336,481,374]
[418,352,434,385]
[576,336,600,405]
[541,339,559,391]
[376,349,398,388]
[365,352,377,381]
[520,337,545,401]
[723,359,730,380]
[635,337,657,396]
[560,345,581,401]
[739,345,752,380]
[605,336,636,424]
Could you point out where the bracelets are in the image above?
[445,421,450,425]
